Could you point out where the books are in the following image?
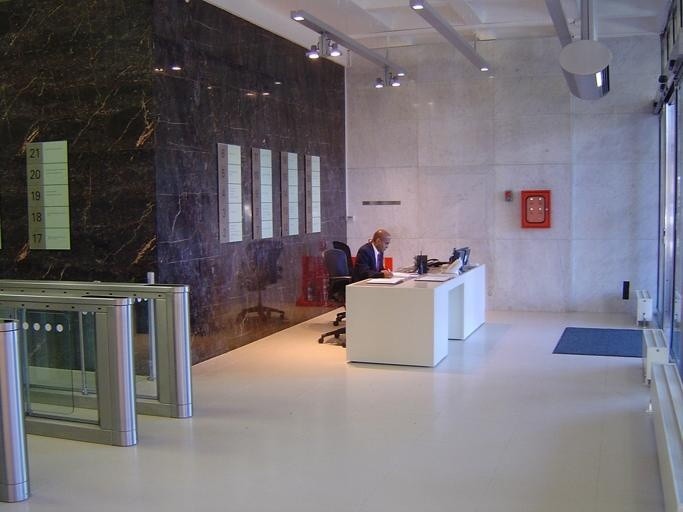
[366,272,419,285]
[415,273,457,282]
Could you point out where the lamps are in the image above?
[305,36,341,61]
[373,63,405,93]
[561,40,613,105]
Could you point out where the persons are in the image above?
[351,229,396,282]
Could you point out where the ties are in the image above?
[377,252,379,273]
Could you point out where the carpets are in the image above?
[552,322,647,359]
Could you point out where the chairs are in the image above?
[235,236,288,327]
[320,238,355,343]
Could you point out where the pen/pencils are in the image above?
[387,268,391,272]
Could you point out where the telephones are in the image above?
[427,259,443,267]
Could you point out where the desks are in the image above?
[343,264,487,369]
